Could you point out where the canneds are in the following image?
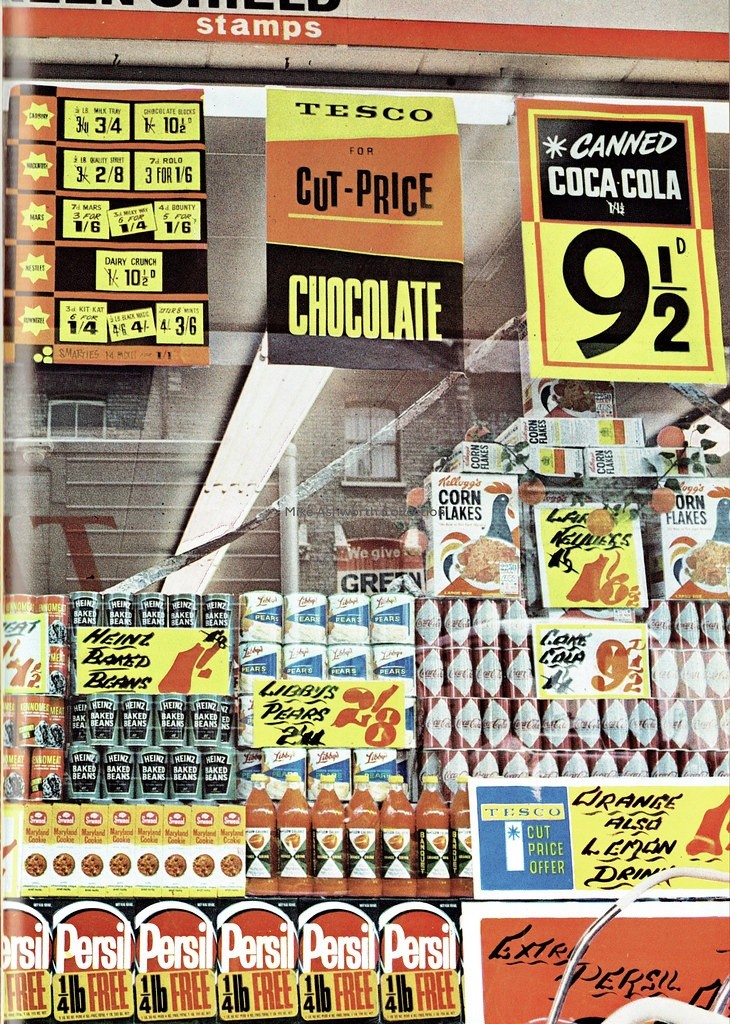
[417,598,730,806]
[3,591,417,805]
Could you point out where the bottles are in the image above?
[451,776,473,898]
[276,773,313,894]
[415,775,450,898]
[343,774,385,898]
[243,772,280,895]
[380,774,419,898]
[313,773,349,896]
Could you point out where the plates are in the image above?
[683,540,728,593]
[454,537,519,590]
